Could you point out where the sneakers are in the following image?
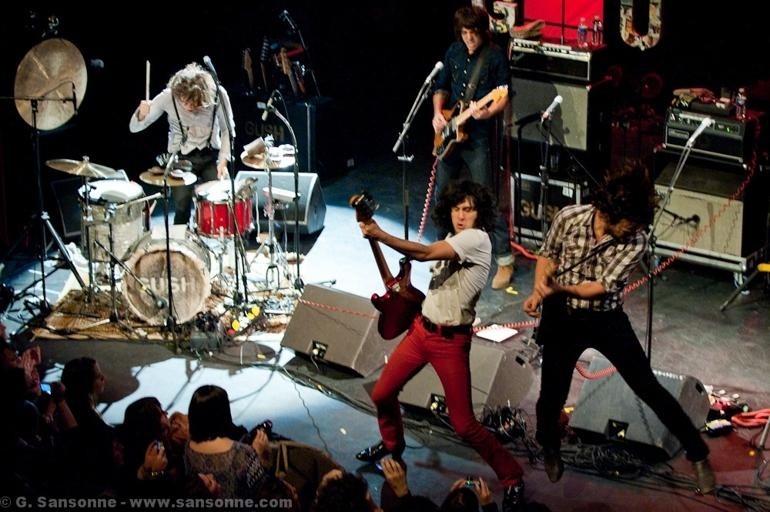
[492,265,514,289]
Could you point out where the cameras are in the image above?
[465,474,480,488]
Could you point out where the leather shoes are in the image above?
[544,441,563,483]
[503,479,526,511]
[355,441,405,461]
[695,458,715,493]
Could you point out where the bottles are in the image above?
[577,17,588,48]
[735,88,747,120]
[590,16,603,47]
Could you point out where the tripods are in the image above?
[14,165,335,339]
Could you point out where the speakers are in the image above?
[280,282,412,377]
[387,338,537,429]
[567,355,711,467]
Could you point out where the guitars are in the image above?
[349,190,426,341]
[534,261,557,345]
[434,84,508,161]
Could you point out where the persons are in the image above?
[521,160,716,497]
[429,6,517,292]
[355,179,525,512]
[127,59,237,226]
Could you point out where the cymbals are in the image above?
[139,170,197,187]
[46,158,116,178]
[240,147,295,169]
[14,39,88,131]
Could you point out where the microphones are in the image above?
[71,89,81,120]
[687,117,713,146]
[539,94,562,122]
[261,90,283,121]
[424,61,445,86]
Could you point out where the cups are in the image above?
[243,136,268,159]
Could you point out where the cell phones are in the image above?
[155,439,163,451]
[39,383,51,394]
[374,458,384,472]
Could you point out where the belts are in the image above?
[421,318,469,338]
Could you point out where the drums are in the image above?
[78,180,144,262]
[196,180,252,238]
[122,224,211,325]
